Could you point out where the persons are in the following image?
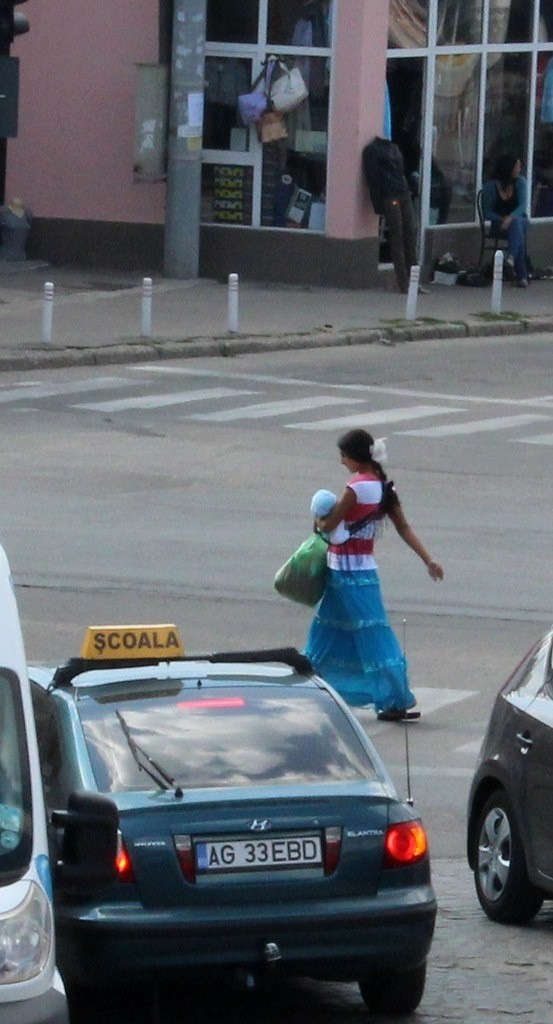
[481,153,531,288]
[309,490,352,545]
[360,136,427,293]
[0,197,34,262]
[295,428,444,721]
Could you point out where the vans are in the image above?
[0,542,69,1023]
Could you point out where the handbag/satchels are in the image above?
[273,528,330,608]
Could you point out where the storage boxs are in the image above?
[212,136,287,226]
[295,129,327,162]
[434,272,458,286]
[308,202,326,230]
[286,188,313,224]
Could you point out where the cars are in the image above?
[22,623,439,1013]
[463,625,553,925]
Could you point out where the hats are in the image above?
[311,489,337,517]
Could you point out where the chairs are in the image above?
[476,189,531,287]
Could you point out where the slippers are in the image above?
[378,707,420,721]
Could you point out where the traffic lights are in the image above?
[1,0,29,50]
